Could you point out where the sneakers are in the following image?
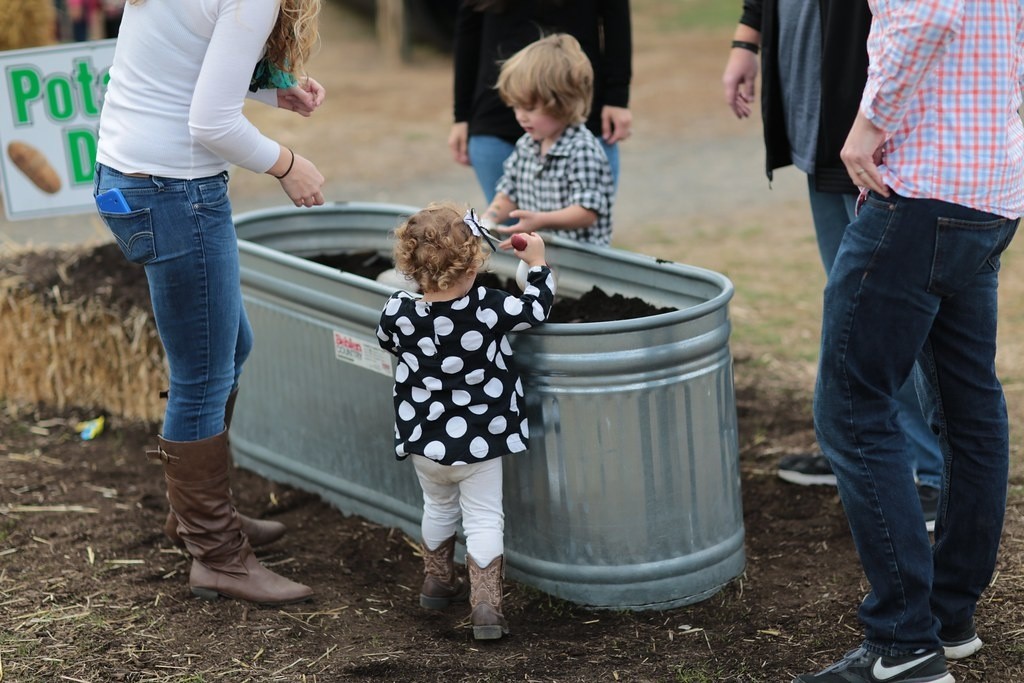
[790,642,957,683]
[935,620,983,660]
[915,481,937,533]
[777,453,919,491]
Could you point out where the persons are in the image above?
[377,202,557,639]
[448,0,632,227]
[723,0,945,532]
[796,1,1024,682]
[481,34,614,248]
[92,1,326,605]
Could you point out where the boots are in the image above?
[159,425,314,606]
[158,385,287,547]
[466,552,509,640]
[421,531,470,610]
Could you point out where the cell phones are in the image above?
[96,187,131,214]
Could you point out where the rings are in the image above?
[857,169,867,175]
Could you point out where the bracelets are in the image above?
[732,41,760,55]
[274,148,294,179]
